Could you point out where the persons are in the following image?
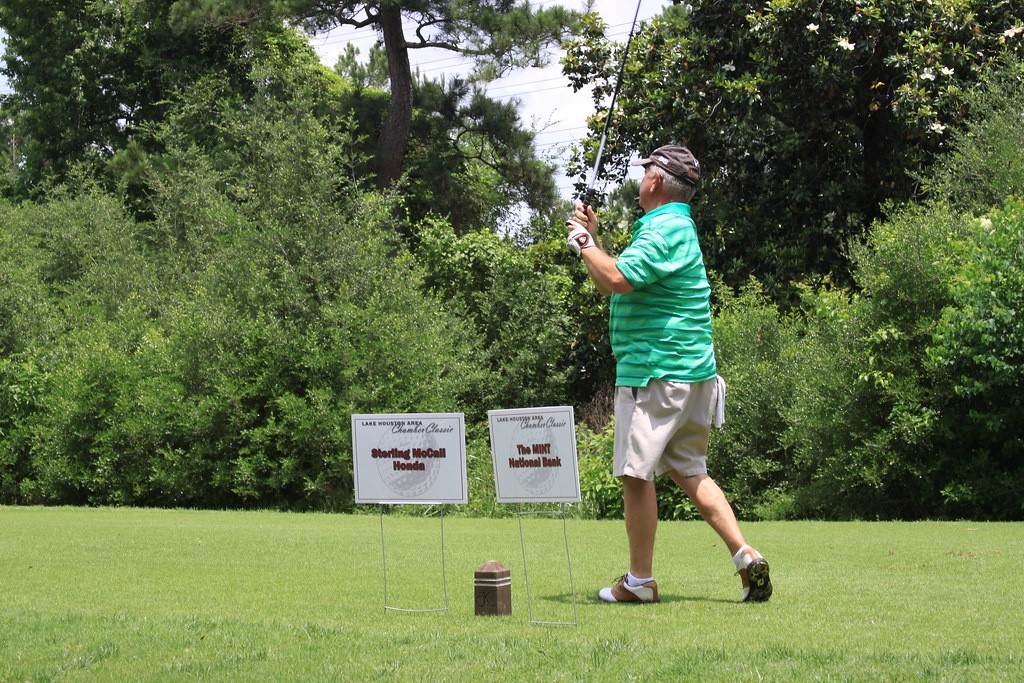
[565,144,773,603]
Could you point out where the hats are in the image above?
[631,144,700,186]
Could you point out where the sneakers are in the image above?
[599,574,659,604]
[735,547,773,603]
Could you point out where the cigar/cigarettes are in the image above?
[634,197,640,199]
